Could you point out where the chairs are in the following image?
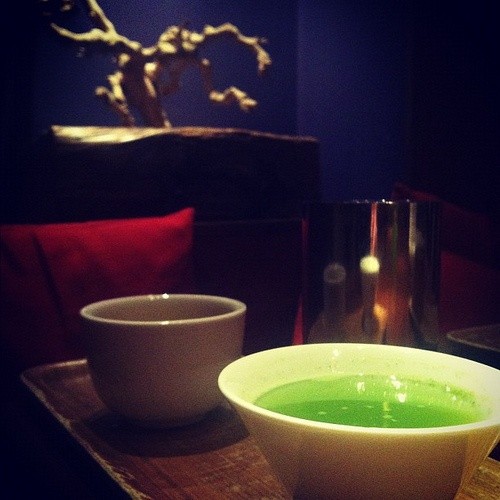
[2,208,195,372]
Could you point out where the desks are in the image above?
[16,336,500,498]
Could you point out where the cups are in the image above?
[79,294,247,429]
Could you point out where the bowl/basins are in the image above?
[217,342,500,499]
